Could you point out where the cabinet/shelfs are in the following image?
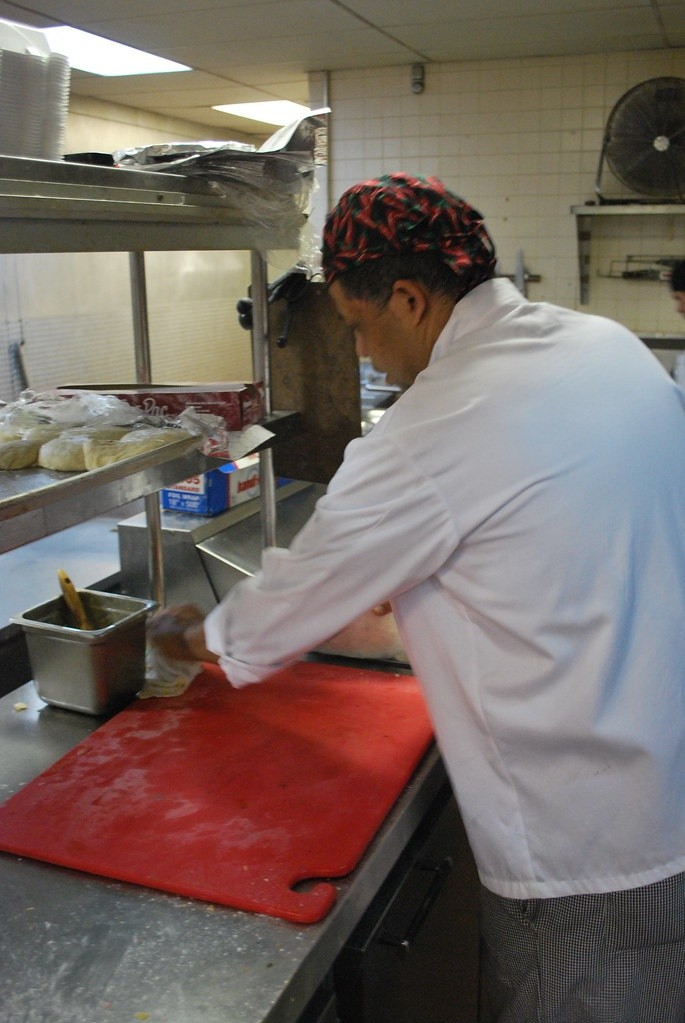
[0,216,307,644]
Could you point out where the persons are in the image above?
[145,173,685,1022]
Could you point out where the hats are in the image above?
[320,171,498,288]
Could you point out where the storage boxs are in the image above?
[161,453,260,518]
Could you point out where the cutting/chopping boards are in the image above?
[0,661,433,923]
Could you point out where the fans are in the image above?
[594,77,685,205]
